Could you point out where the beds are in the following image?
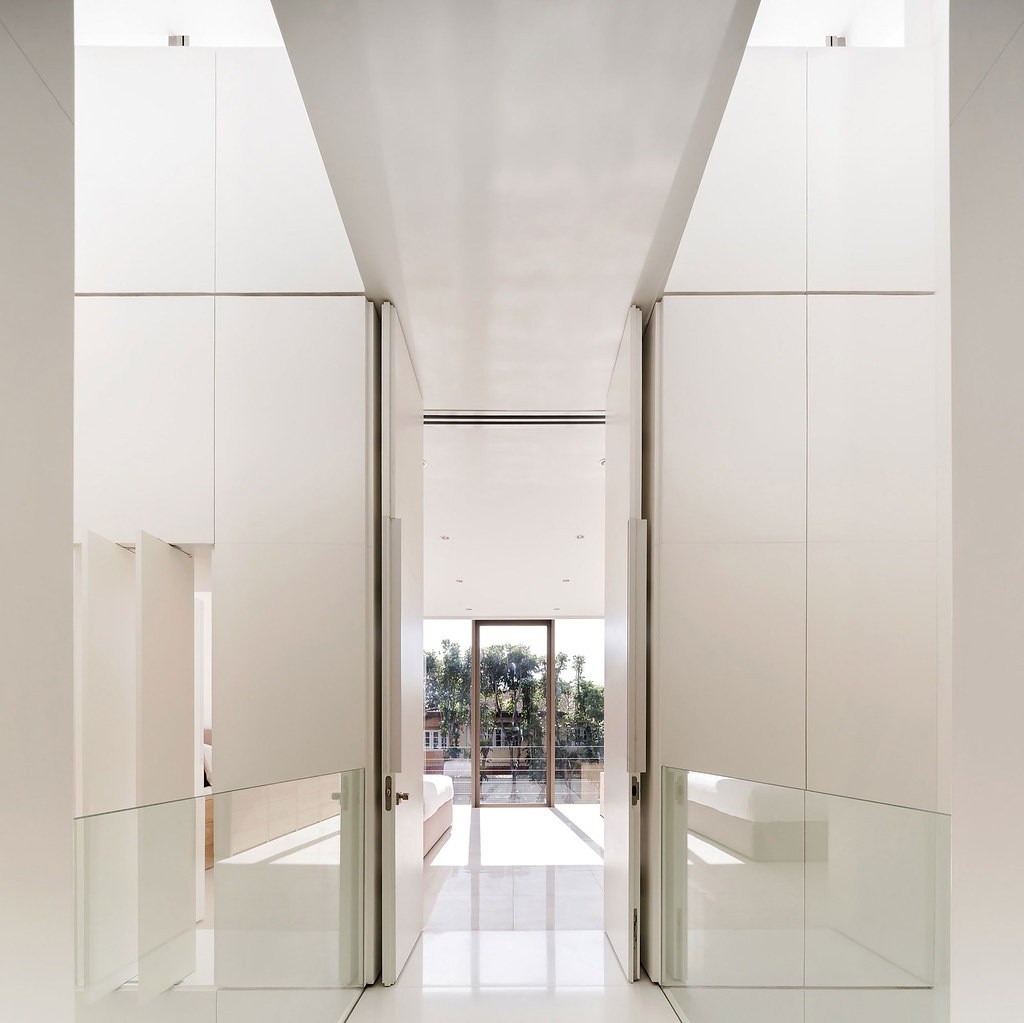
[423,775,455,859]
